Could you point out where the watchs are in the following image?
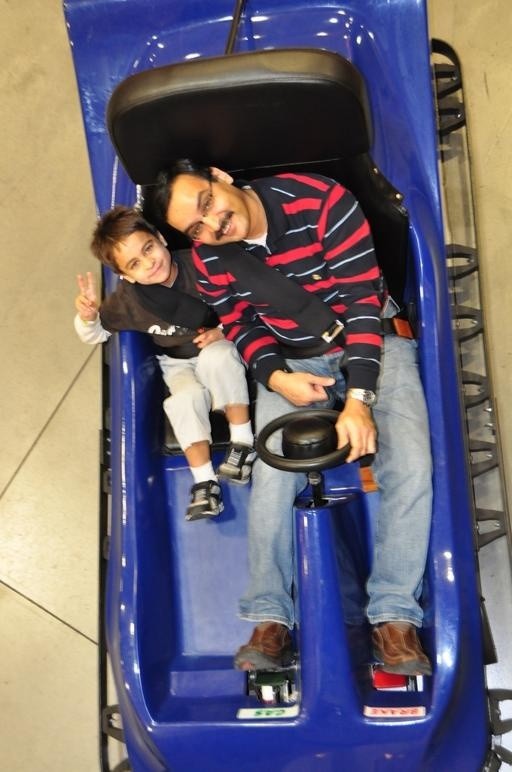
[348,384,377,408]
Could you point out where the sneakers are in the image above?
[233,619,297,674]
[214,441,258,486]
[184,478,226,524]
[370,618,433,677]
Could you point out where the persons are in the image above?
[70,204,258,523]
[140,152,434,678]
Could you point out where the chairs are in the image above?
[101,48,428,450]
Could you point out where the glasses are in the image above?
[186,172,216,243]
